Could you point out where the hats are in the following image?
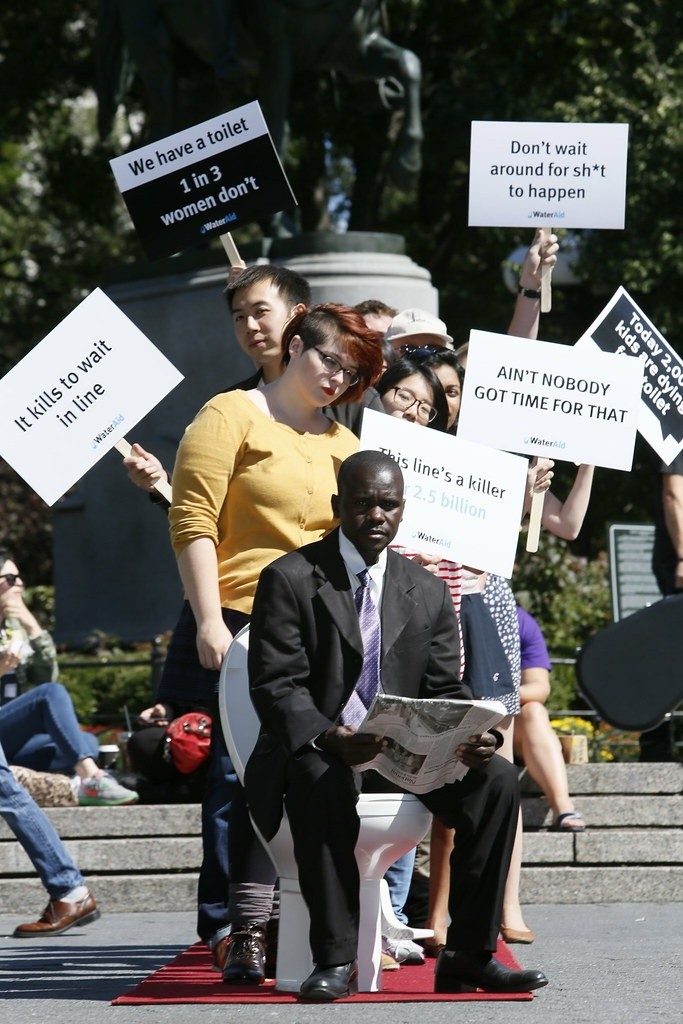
[384,307,454,348]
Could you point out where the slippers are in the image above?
[550,810,586,832]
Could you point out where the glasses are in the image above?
[310,343,360,387]
[393,344,445,355]
[380,379,438,422]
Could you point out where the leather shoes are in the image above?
[299,960,358,1001]
[433,947,549,994]
[12,890,101,937]
[221,920,267,985]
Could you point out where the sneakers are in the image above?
[77,768,138,806]
[380,933,426,965]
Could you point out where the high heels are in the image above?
[500,923,535,944]
[421,922,447,957]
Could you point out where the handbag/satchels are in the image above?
[161,709,213,775]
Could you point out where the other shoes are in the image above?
[381,953,399,970]
[215,936,232,969]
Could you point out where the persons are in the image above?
[250,450,547,1000]
[1,228,683,981]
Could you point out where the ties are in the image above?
[338,570,381,728]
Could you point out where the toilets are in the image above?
[217,622,433,993]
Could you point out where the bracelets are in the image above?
[677,558,683,562]
[517,282,542,299]
